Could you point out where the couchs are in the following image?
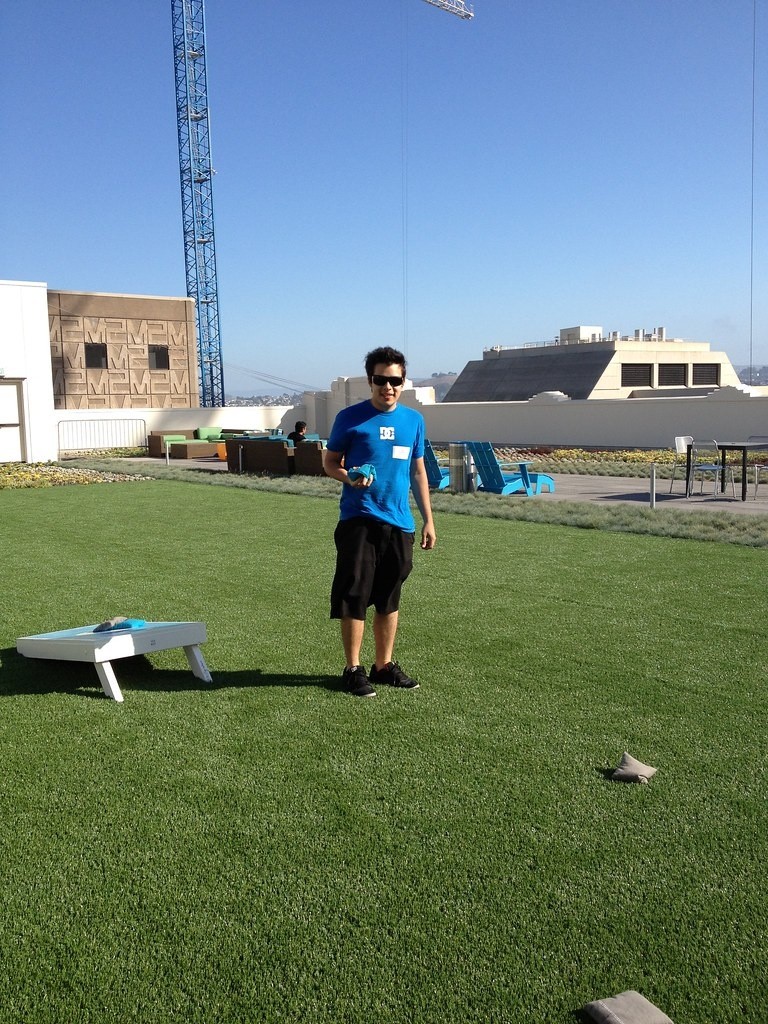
[148,426,345,477]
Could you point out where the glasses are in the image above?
[371,375,403,386]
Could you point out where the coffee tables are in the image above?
[170,444,221,459]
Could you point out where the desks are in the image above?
[685,442,768,501]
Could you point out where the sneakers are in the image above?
[343,666,377,697]
[369,661,420,690]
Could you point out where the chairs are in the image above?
[669,436,720,496]
[424,439,484,490]
[741,435,768,500]
[689,438,736,500]
[467,440,554,497]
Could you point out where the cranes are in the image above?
[170,1,477,408]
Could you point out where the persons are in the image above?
[324,348,437,698]
[287,421,307,447]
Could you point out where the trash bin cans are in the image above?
[447,440,478,493]
